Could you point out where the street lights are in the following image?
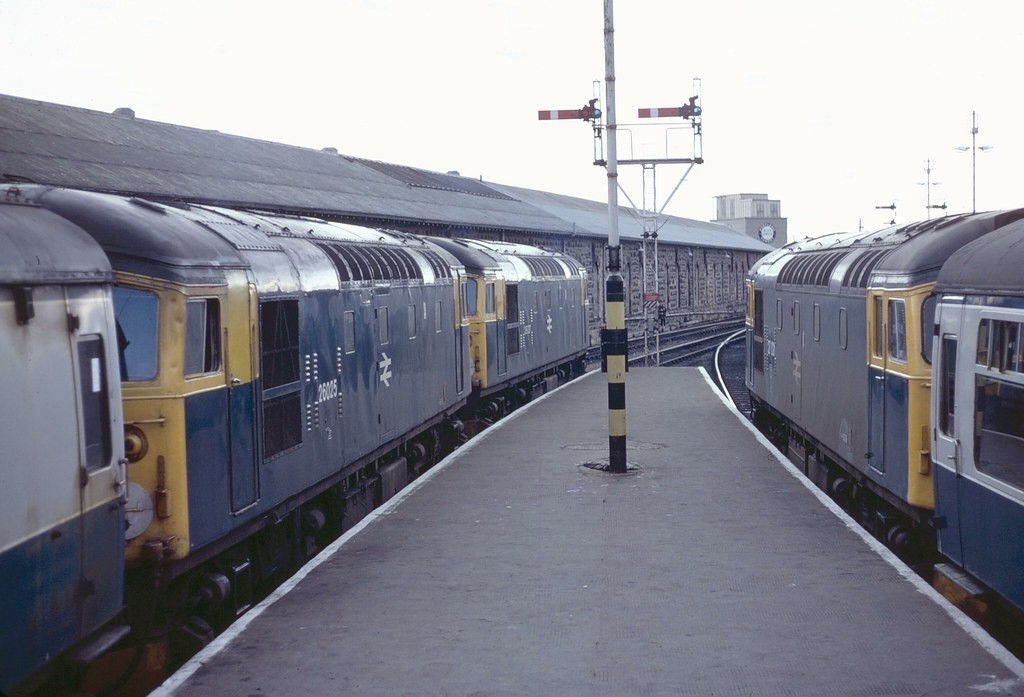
[914,157,945,218]
[954,110,995,214]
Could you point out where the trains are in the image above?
[0,184,593,693]
[744,208,1024,619]
